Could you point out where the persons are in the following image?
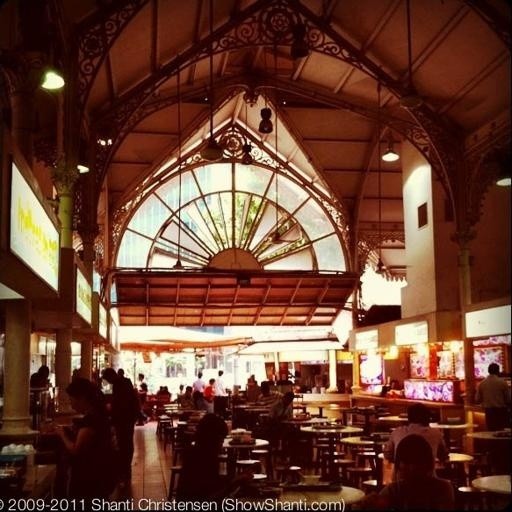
[351,433,456,509]
[30,364,295,510]
[476,362,511,431]
[383,403,455,471]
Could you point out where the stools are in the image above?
[156,404,512,512]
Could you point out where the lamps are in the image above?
[382,129,400,162]
[241,103,252,165]
[399,0,424,110]
[259,97,273,133]
[200,0,224,161]
[291,0,310,58]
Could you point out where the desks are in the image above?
[0,463,59,511]
